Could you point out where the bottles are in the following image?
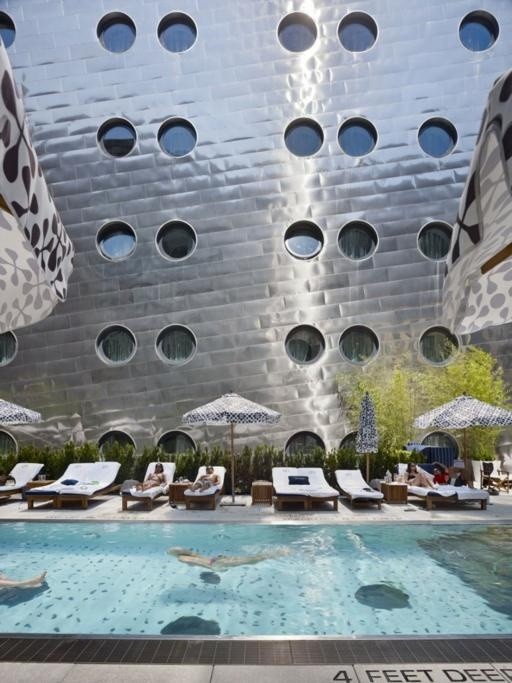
[394,473,398,481]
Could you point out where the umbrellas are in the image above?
[0,398,41,427]
[410,391,512,470]
[354,390,378,486]
[1,39,76,343]
[442,67,512,339]
[182,391,285,506]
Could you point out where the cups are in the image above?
[179,477,183,482]
[384,475,390,482]
[38,474,46,481]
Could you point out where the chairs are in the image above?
[0,456,512,514]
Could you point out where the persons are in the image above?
[432,467,476,489]
[0,569,50,589]
[186,464,221,493]
[136,462,168,493]
[168,546,293,572]
[403,462,440,490]
